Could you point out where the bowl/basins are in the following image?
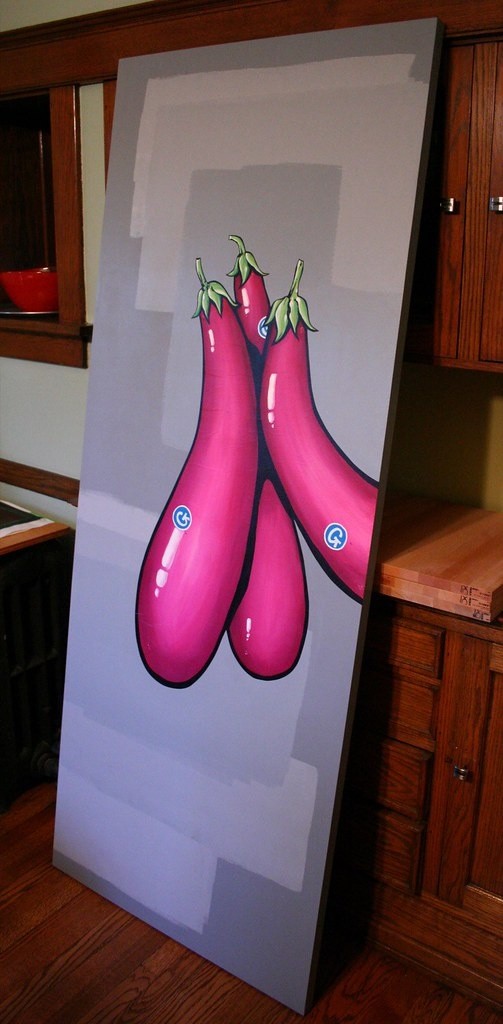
[0,267,59,312]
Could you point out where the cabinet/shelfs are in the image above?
[404,35,503,373]
[326,608,503,1013]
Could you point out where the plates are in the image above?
[0,312,59,318]
